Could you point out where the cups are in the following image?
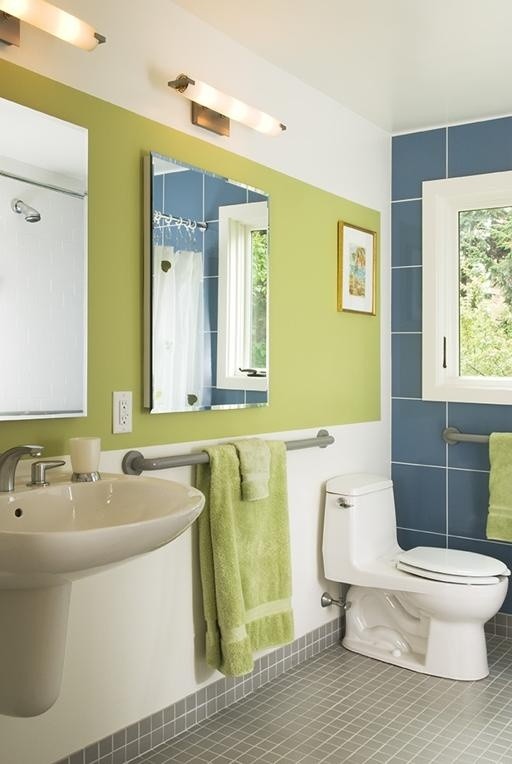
[66,436,103,482]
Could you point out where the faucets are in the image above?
[0,444,45,492]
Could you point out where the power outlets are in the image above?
[112,390,133,434]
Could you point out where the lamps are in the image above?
[0,0,108,55]
[169,72,287,140]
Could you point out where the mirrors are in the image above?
[142,151,269,416]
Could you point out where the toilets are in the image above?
[321,472,512,680]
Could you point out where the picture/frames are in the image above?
[339,220,378,315]
[1,173,92,421]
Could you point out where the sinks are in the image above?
[0,472,206,591]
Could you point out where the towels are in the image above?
[487,431,512,545]
[196,439,296,677]
